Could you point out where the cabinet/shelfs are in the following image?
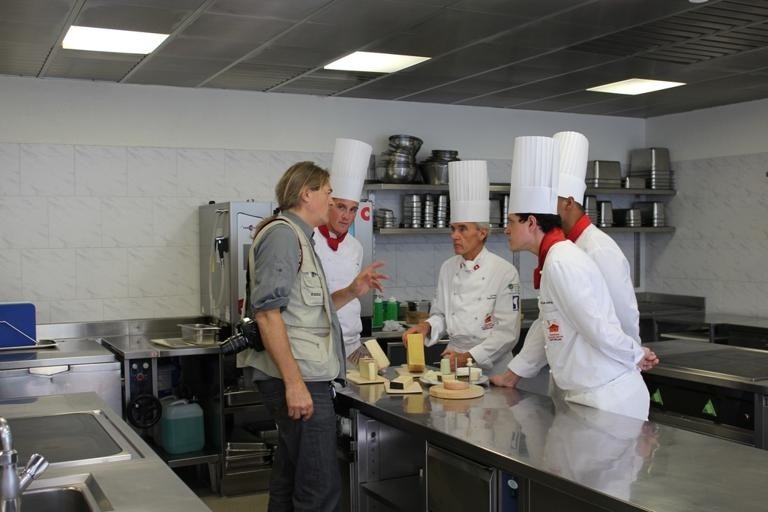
[367,182,677,234]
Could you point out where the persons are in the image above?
[402,221,521,382]
[490,212,658,422]
[547,196,643,396]
[309,198,374,367]
[237,160,390,512]
[432,388,660,501]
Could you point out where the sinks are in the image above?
[0,473,116,512]
[0,409,145,469]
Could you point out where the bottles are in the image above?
[373,296,397,328]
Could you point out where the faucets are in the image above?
[0,417,49,500]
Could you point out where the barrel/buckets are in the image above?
[160,397,206,454]
[154,394,179,439]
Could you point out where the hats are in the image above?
[329,138,373,203]
[508,131,589,215]
[447,160,490,223]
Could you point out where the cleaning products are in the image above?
[384,295,397,322]
[371,294,385,330]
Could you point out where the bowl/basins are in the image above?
[374,134,462,184]
[373,193,449,229]
